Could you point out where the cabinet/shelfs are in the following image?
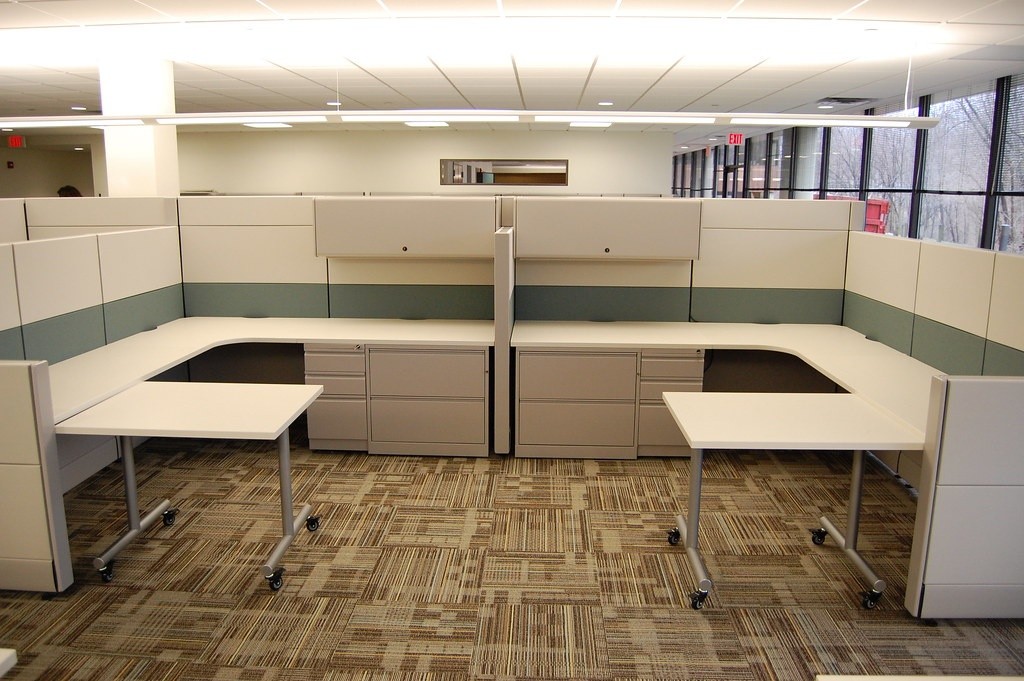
[302,343,489,458]
[514,347,706,461]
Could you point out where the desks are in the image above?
[54,380,325,591]
[662,391,928,616]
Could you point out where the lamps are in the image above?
[0,57,943,135]
